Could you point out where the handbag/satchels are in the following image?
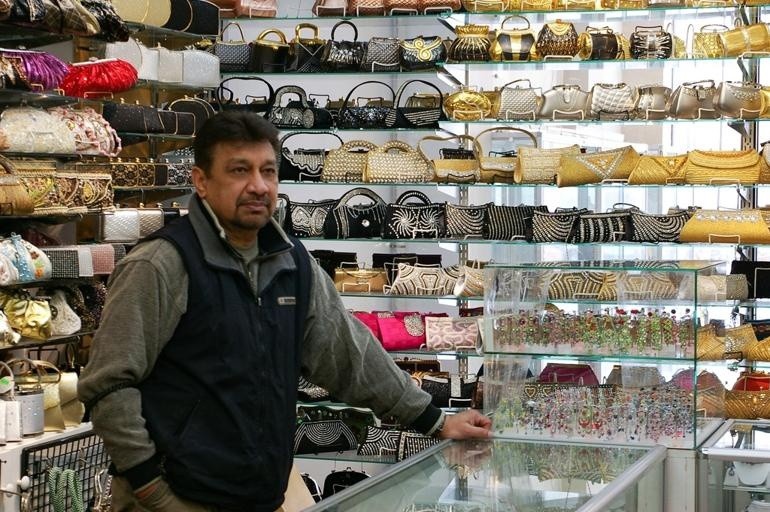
[220,74,493,129]
[0,0,221,245]
[347,303,518,351]
[295,374,443,463]
[0,246,127,443]
[274,187,531,243]
[277,128,538,185]
[309,250,766,299]
[438,0,767,62]
[221,0,277,19]
[530,202,766,245]
[218,20,438,73]
[393,357,765,419]
[538,143,766,187]
[312,0,438,15]
[492,78,765,121]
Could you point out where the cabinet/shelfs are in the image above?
[1,0,769,512]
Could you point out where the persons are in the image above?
[77,107,496,512]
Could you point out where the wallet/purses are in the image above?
[695,318,766,360]
